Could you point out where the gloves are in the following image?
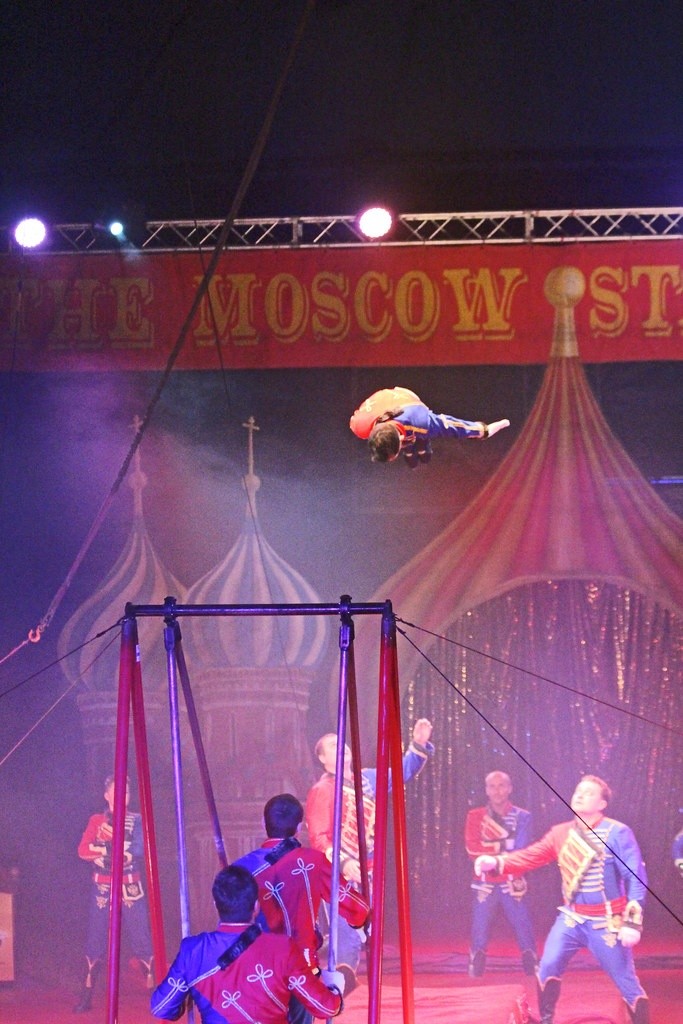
[487,419,510,439]
[356,922,371,943]
[474,855,497,876]
[320,968,345,995]
[616,927,642,947]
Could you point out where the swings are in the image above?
[162,614,371,1024]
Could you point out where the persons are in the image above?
[672,807,682,880]
[151,864,345,1024]
[348,384,512,472]
[463,770,541,976]
[307,717,436,968]
[72,772,158,1016]
[225,793,371,1023]
[473,774,649,1024]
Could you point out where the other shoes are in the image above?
[403,453,419,469]
[416,447,433,463]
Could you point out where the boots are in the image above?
[71,954,101,1012]
[532,966,561,1024]
[139,956,156,996]
[467,947,488,978]
[620,996,651,1024]
[522,949,536,975]
